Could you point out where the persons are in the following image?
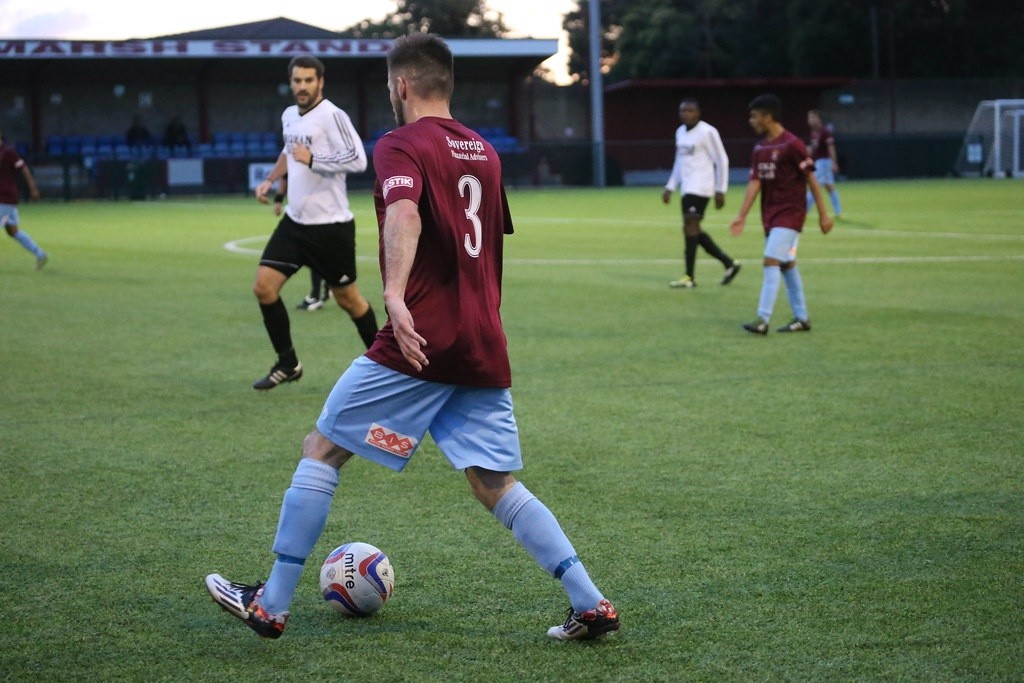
[663,96,741,288]
[805,108,840,217]
[206,35,620,640]
[731,94,833,335]
[126,115,157,145]
[0,133,48,270]
[162,115,192,158]
[253,54,378,390]
[274,89,332,310]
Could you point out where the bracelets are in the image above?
[274,193,283,204]
[308,154,314,168]
[266,177,273,183]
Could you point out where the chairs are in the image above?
[48,125,529,202]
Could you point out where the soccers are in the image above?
[319,542,395,616]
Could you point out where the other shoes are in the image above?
[295,294,330,310]
[835,215,846,222]
[36,253,48,270]
[671,276,695,289]
[721,260,740,286]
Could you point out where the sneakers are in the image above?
[253,360,302,390]
[205,574,290,639]
[743,318,769,334]
[777,316,811,332]
[547,599,619,640]
